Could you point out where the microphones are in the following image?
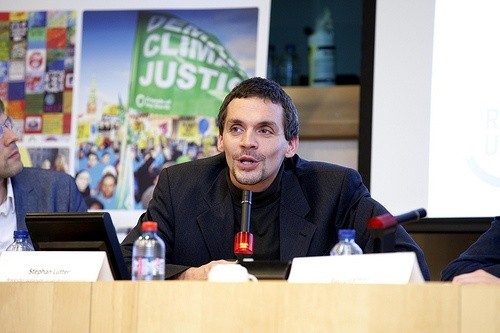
[232,189,255,257]
[369,209,428,231]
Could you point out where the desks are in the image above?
[1,282,498,333]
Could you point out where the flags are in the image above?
[130,10,249,116]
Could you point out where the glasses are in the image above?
[0,116,13,139]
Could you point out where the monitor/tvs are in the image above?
[26,212,132,281]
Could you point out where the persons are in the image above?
[119,76,431,281]
[440,217,500,285]
[41,154,67,174]
[75,113,221,210]
[0,100,88,251]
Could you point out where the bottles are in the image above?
[131,221,166,280]
[330,229,362,255]
[6,230,35,251]
